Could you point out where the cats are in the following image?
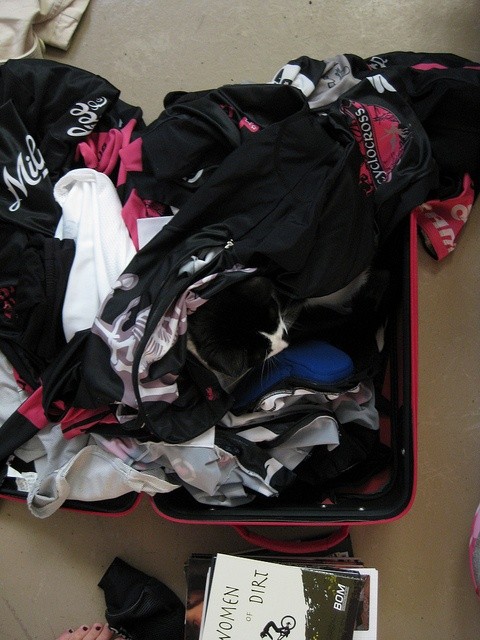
[190,270,377,380]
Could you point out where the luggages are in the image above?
[0,212,419,554]
